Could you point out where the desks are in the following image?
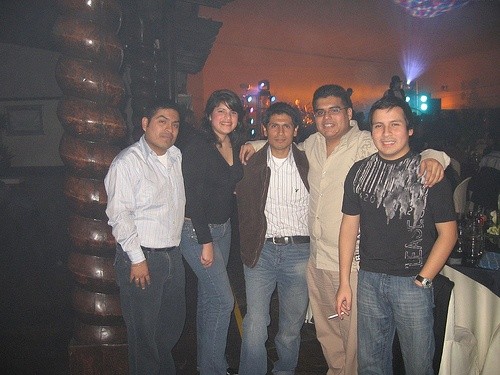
[440,241,500,372]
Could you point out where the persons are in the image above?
[239,85,451,375]
[384,75,405,100]
[235,103,312,375]
[180,89,243,375]
[345,87,353,106]
[104,99,186,375]
[335,96,458,375]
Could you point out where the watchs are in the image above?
[415,274,433,289]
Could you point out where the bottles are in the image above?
[455,217,465,253]
[463,216,479,265]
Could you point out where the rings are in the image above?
[135,277,140,282]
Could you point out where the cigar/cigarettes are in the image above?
[328,311,344,320]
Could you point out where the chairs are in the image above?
[453,176,472,213]
